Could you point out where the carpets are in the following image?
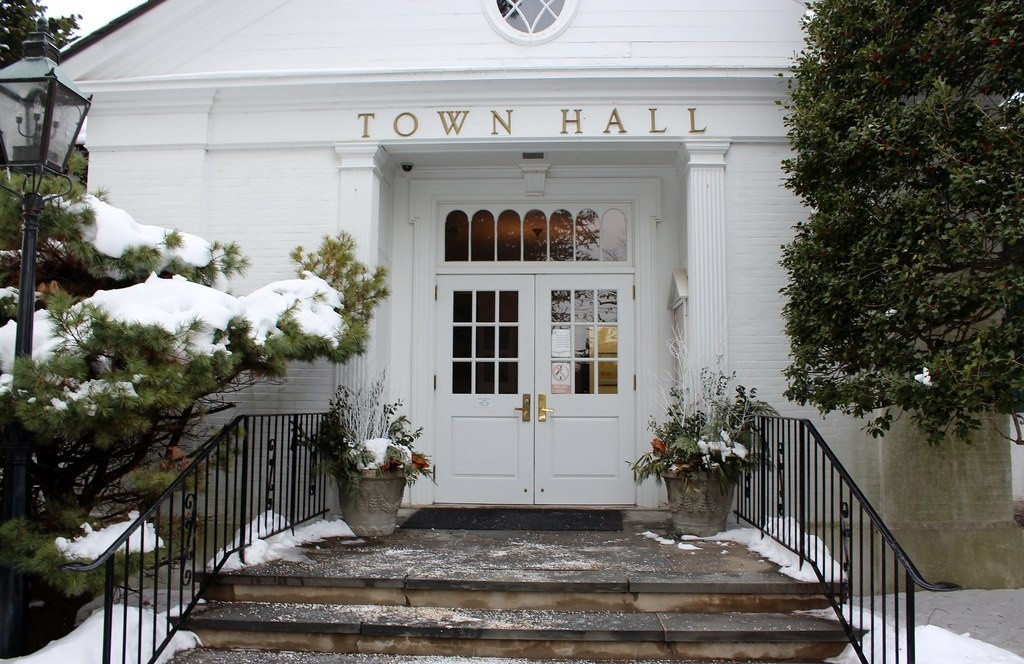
[397,507,624,532]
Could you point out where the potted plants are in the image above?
[288,371,435,537]
[624,323,786,537]
